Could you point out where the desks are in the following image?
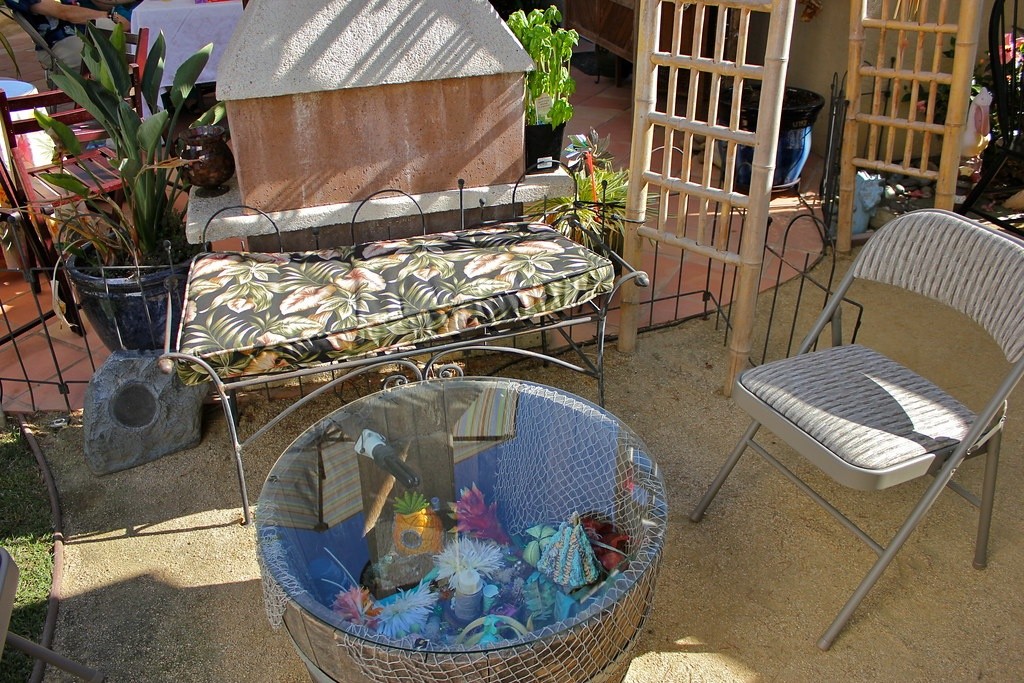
[130,0,243,145]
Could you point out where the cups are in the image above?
[178,127,235,197]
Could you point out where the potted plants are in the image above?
[527,123,665,277]
[506,4,578,174]
[32,19,220,347]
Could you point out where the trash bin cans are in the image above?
[249,383,669,683]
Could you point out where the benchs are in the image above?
[156,213,651,528]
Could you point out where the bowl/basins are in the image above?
[716,84,825,191]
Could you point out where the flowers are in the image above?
[900,26,1024,213]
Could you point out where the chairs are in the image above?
[9,8,79,115]
[0,64,142,336]
[953,0,1024,237]
[694,213,1024,650]
